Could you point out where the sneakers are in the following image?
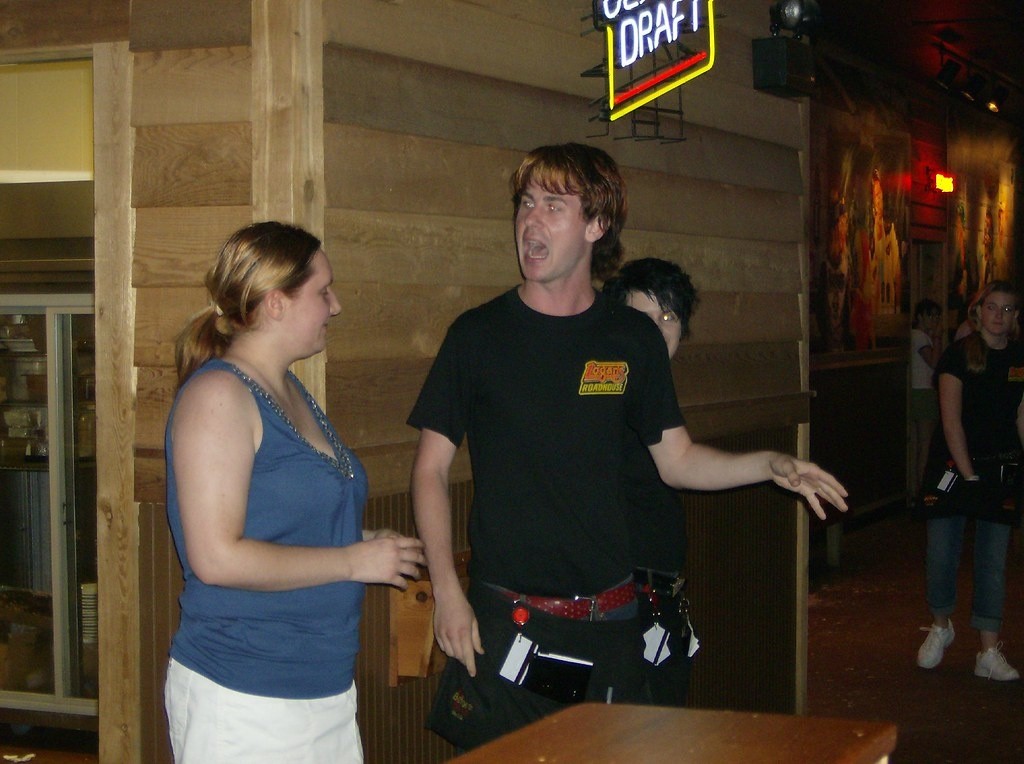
[974,640,1020,681]
[916,618,956,668]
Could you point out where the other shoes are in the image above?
[913,494,938,507]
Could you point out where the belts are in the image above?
[498,582,634,621]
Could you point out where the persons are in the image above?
[906,279,1023,682]
[408,141,847,755]
[165,220,427,763]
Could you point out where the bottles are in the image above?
[79,375,95,400]
[77,404,96,457]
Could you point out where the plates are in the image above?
[78,583,99,645]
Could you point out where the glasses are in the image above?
[654,311,680,327]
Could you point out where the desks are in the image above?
[442,702,899,764]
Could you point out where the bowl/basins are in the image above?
[0,339,38,352]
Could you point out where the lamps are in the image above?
[937,44,1010,113]
[753,1,821,99]
